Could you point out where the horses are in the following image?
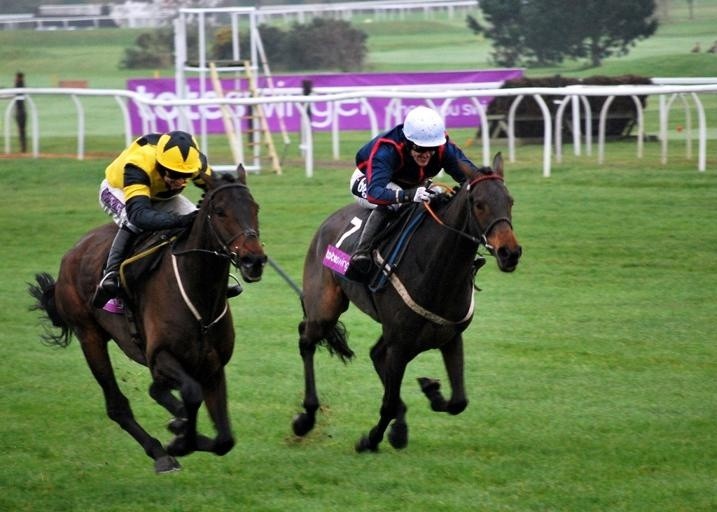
[25,162,269,475]
[290,151,523,454]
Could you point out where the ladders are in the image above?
[209,60,282,176]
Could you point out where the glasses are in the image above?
[167,171,194,180]
[407,145,436,153]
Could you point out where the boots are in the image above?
[344,204,390,280]
[91,229,136,309]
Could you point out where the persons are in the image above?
[94,131,217,316]
[348,104,482,271]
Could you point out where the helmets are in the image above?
[156,130,208,173]
[401,104,447,148]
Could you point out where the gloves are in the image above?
[404,178,437,203]
[176,209,198,229]
